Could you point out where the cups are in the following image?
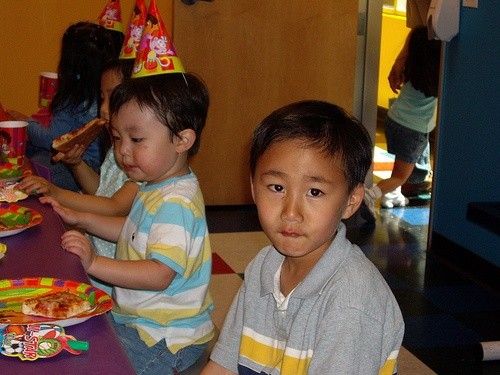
[0,121,29,168]
[38,73,57,107]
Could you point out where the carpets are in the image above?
[208,232,436,375]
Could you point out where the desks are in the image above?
[0,161,137,375]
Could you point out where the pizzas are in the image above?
[22,291,91,319]
[52,117,106,154]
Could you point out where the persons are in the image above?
[363,0,445,218]
[198,100,405,375]
[38,70,215,375]
[18,58,142,296]
[5,21,125,196]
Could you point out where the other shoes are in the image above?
[379,185,409,208]
[363,187,376,219]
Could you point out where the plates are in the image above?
[0,164,33,182]
[0,277,114,326]
[0,203,44,236]
